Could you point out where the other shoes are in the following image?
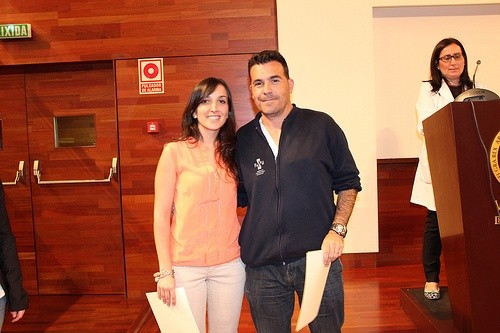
[424,281,440,299]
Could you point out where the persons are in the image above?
[0,174,31,333]
[153,77,246,333]
[410,38,473,301]
[233,49,362,333]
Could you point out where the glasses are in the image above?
[439,53,464,63]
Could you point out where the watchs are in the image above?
[330,223,347,238]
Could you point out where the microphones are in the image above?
[473,60,481,88]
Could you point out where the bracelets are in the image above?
[154,270,174,282]
